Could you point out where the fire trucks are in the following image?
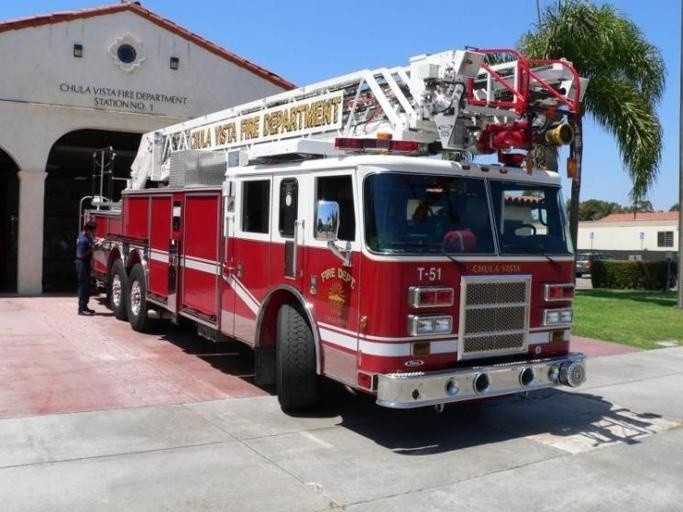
[78,45,590,419]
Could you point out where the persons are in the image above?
[73,221,98,316]
[408,205,429,234]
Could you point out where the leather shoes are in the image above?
[80,310,94,316]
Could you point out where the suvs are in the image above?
[575,252,609,278]
